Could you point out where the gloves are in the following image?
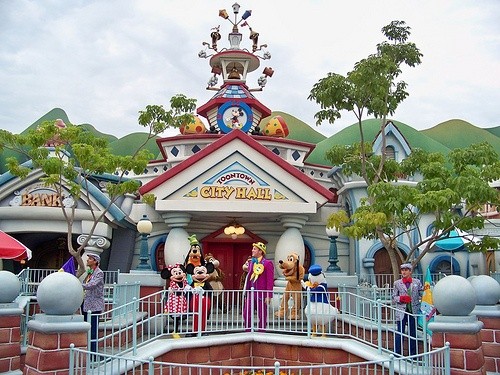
[400,296,411,303]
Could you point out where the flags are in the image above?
[58,257,76,277]
[418,266,436,337]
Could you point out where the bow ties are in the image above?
[251,257,258,264]
[402,277,412,283]
[87,269,94,275]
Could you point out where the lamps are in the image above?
[137,215,152,237]
[326,224,340,237]
[224,217,245,239]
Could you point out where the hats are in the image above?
[87,254,101,263]
[252,242,266,254]
[400,263,413,270]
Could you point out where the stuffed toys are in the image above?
[239,253,305,320]
[301,265,335,338]
[161,234,225,338]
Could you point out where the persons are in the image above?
[393,264,424,362]
[242,242,274,332]
[80,254,105,367]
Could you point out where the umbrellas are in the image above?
[0,230,32,262]
[417,220,482,275]
